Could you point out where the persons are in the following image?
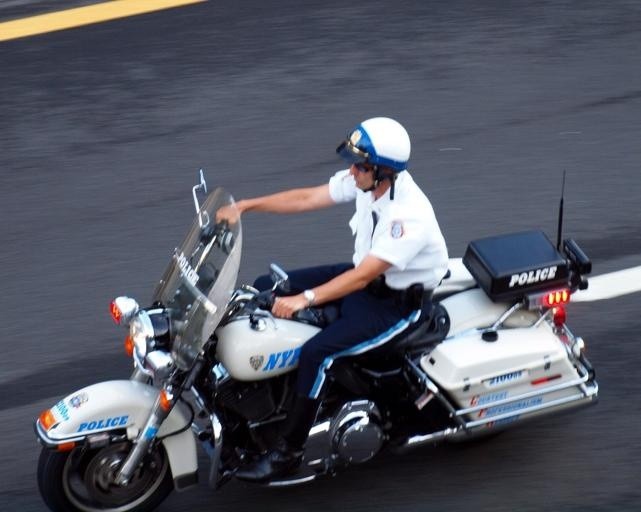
[210,114,452,483]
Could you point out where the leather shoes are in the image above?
[233,447,305,486]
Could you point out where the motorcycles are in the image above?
[33,168,600,511]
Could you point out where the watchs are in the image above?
[303,289,317,308]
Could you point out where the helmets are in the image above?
[335,115,411,171]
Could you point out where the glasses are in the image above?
[353,162,377,173]
